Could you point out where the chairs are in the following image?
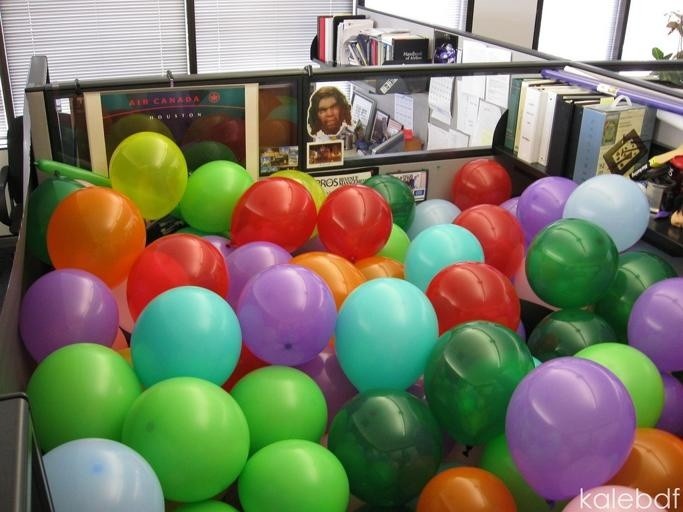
[1,114,75,236]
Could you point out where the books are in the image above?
[318,14,430,66]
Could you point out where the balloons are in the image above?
[16,129,683,512]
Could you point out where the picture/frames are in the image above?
[350,90,377,141]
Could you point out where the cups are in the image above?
[340,132,353,150]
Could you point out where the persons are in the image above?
[307,87,352,135]
